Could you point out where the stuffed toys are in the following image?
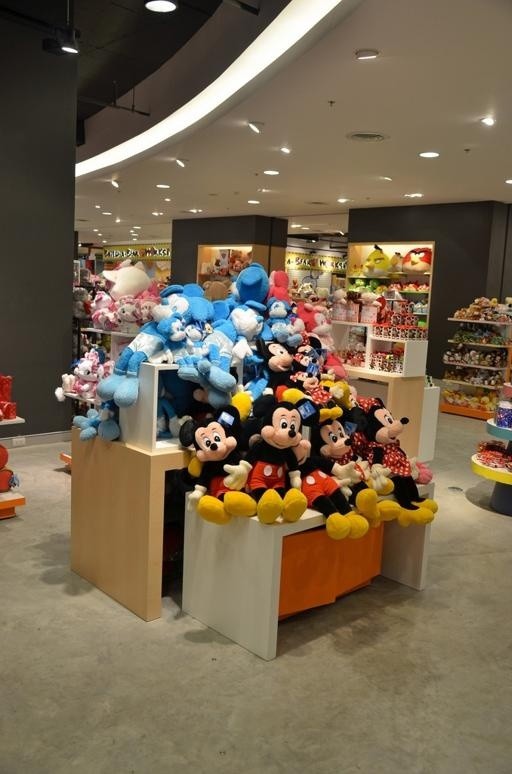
[54,245,432,440]
[443,297,509,410]
[173,403,256,524]
[349,395,436,526]
[53,245,509,441]
[290,434,373,538]
[300,397,400,521]
[243,394,312,524]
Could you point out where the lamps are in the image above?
[174,157,185,170]
[249,121,264,132]
[146,1,176,17]
[59,1,81,55]
[111,179,121,187]
[354,47,379,60]
